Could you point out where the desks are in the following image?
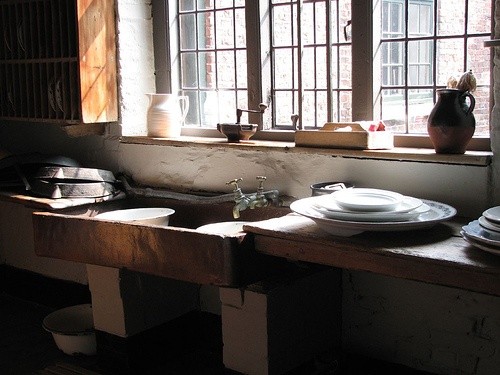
[243,206,500,298]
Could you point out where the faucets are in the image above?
[248,175,269,210]
[224,177,257,219]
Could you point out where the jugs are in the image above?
[144,92,189,140]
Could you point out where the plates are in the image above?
[460,205,500,254]
[289,188,458,237]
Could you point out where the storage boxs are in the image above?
[295,123,396,151]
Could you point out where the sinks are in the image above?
[31,189,292,288]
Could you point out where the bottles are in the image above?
[426,89,476,155]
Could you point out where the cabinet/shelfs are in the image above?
[0,0,119,139]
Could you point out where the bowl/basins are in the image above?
[311,181,355,195]
[41,303,97,356]
[93,207,175,227]
